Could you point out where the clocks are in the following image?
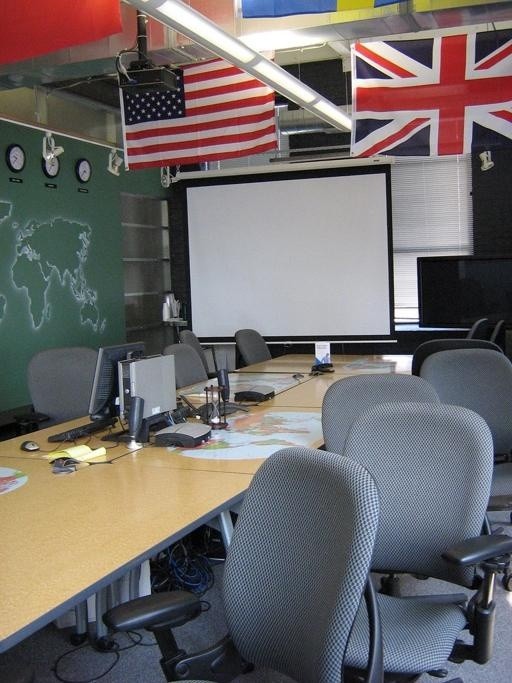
[42,153,61,178]
[73,157,94,185]
[5,143,27,172]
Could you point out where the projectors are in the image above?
[118,67,177,90]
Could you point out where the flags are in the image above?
[113,52,282,172]
[240,0,412,19]
[346,27,512,160]
[1,0,123,64]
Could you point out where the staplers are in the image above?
[311,363,335,372]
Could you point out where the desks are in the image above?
[0,350,413,665]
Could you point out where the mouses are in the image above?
[21,441,39,451]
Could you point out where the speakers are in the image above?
[125,396,143,450]
[217,369,248,412]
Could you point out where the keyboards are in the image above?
[48,417,118,442]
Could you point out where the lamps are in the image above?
[120,0,355,135]
[32,129,176,187]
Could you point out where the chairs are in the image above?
[419,349,512,526]
[411,337,504,377]
[343,400,512,683]
[162,341,212,391]
[179,330,213,377]
[466,317,506,351]
[100,444,388,683]
[235,329,272,367]
[14,345,104,437]
[318,370,512,593]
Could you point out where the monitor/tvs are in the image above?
[88,341,146,442]
[417,255,512,328]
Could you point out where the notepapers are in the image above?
[48,445,106,464]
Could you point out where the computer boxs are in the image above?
[117,354,177,425]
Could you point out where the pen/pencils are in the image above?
[180,395,197,411]
